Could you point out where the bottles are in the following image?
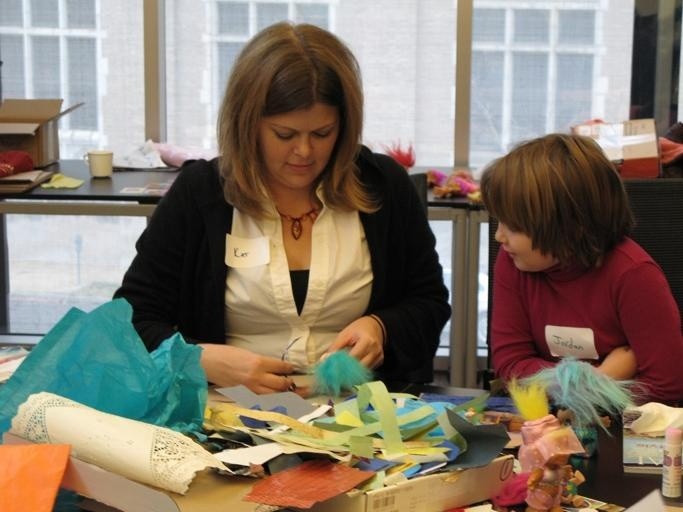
[660,427,683,499]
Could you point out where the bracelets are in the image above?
[368,314,385,347]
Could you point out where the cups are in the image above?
[83,150,114,180]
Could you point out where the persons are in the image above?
[479,133,683,406]
[112,20,452,398]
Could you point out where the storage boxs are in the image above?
[573,118,663,178]
[0,97,87,168]
[5,422,516,511]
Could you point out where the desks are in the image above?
[0,353,682,510]
[1,158,473,388]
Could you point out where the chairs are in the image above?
[482,176,680,398]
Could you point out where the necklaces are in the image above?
[274,205,318,240]
